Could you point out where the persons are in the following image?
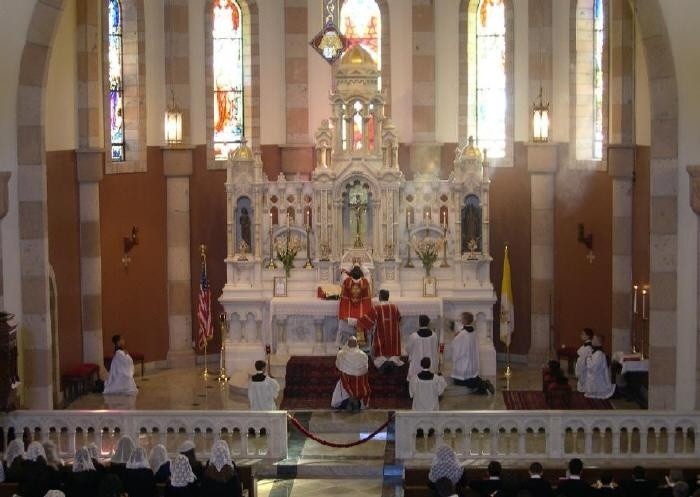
[247,360,280,435]
[0,435,236,497]
[584,334,624,399]
[427,444,700,497]
[446,312,495,395]
[102,335,139,394]
[575,328,594,393]
[331,261,448,411]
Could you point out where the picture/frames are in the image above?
[272,275,288,297]
[422,275,437,297]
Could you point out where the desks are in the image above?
[610,350,648,400]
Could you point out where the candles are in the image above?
[426,215,429,227]
[443,215,446,228]
[287,215,289,228]
[408,215,411,224]
[633,290,637,311]
[642,294,646,319]
[306,215,310,226]
[270,216,273,226]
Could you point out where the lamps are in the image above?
[531,0,552,145]
[162,1,185,148]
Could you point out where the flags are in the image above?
[194,259,213,352]
[500,250,514,348]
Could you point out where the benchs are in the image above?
[63,363,101,398]
[104,350,146,376]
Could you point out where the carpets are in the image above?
[502,387,617,410]
[279,354,414,409]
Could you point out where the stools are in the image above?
[556,346,578,375]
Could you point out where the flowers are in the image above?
[274,233,301,277]
[406,234,444,274]
